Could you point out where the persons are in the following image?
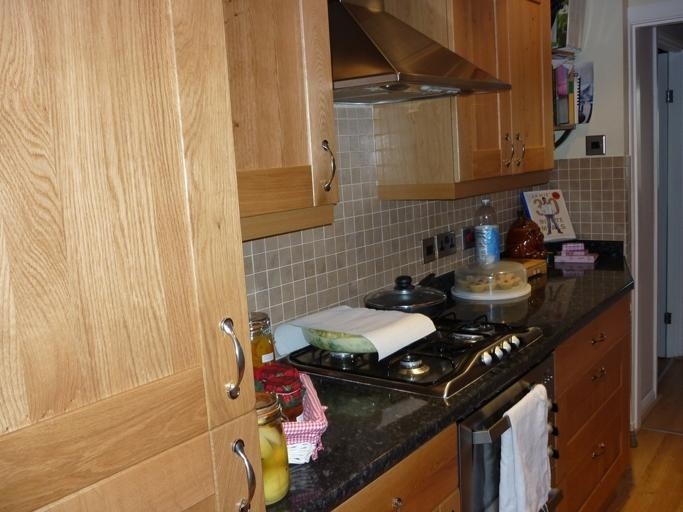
[540,195,563,234]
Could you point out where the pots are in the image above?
[363,271,450,321]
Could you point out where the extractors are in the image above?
[327,0,513,107]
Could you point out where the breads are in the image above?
[457,273,521,293]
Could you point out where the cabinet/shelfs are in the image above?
[324,423,464,512]
[549,291,636,512]
[223,1,340,243]
[369,1,557,202]
[3,0,271,512]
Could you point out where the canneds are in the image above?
[249,310,304,506]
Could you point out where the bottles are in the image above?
[473,197,501,266]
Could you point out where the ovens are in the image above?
[455,353,564,512]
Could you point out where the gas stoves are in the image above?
[286,311,544,401]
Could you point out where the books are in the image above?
[520,189,577,243]
